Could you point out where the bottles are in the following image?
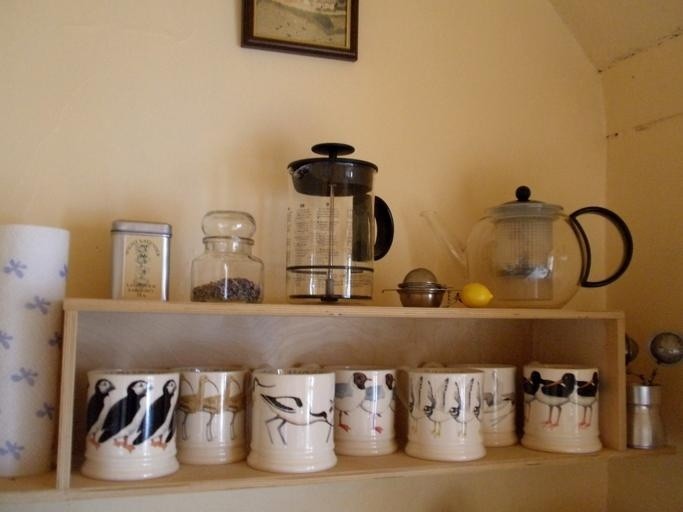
[191,211,265,303]
[628,384,666,449]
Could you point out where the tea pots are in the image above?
[420,185,634,307]
[285,144,394,303]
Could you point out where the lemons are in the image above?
[458,283,494,307]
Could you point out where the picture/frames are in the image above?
[240,0,359,62]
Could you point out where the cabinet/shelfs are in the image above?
[56,296,628,493]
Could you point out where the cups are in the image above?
[337,366,398,456]
[521,362,603,454]
[483,364,518,447]
[81,366,180,481]
[180,370,248,465]
[248,368,339,475]
[398,368,486,463]
